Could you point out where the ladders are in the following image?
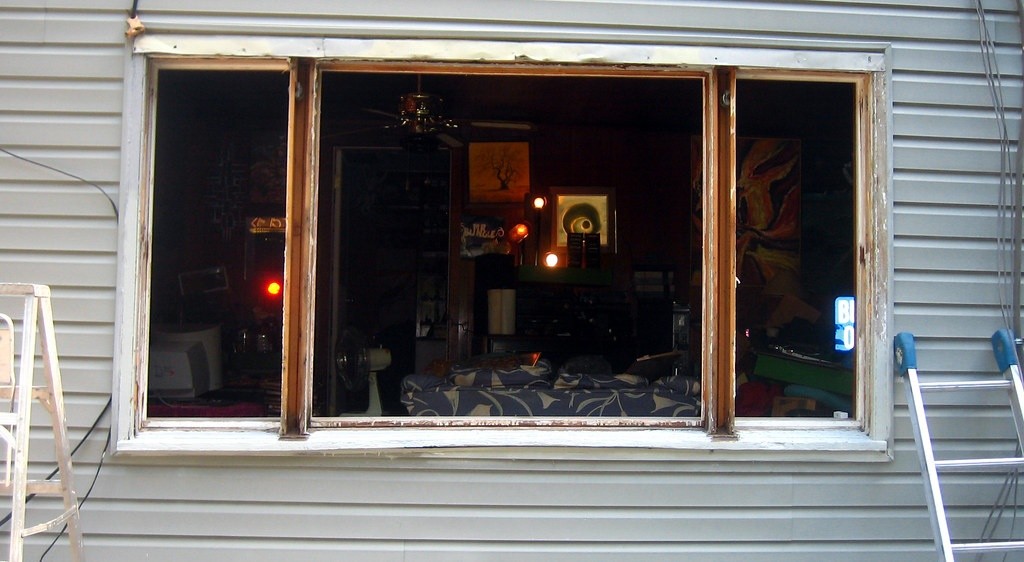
[893,327,1023,561]
[0,279,84,562]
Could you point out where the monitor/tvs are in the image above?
[149,322,223,403]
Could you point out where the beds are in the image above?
[399,353,703,417]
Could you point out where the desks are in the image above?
[147,398,266,417]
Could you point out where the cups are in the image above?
[256,327,274,356]
[232,329,252,354]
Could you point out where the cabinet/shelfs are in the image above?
[414,219,448,376]
[374,170,452,210]
[488,294,638,358]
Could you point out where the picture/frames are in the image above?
[463,136,535,210]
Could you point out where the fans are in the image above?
[320,72,537,195]
[335,325,392,418]
